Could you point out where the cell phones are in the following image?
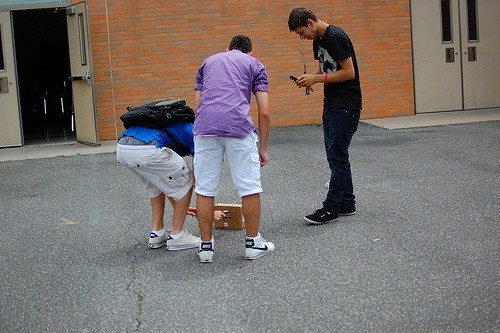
[290,76,298,81]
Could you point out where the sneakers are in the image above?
[244,233,276,261]
[332,202,357,218]
[148,227,171,249]
[164,231,202,252]
[197,237,217,264]
[303,207,340,227]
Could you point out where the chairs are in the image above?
[41,81,67,142]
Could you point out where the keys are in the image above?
[305,86,313,95]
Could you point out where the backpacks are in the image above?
[119,99,195,155]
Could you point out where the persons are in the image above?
[288,7,362,224]
[116,121,226,250]
[193,34,275,262]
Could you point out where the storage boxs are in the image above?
[214,203,246,230]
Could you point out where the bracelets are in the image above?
[325,74,328,84]
